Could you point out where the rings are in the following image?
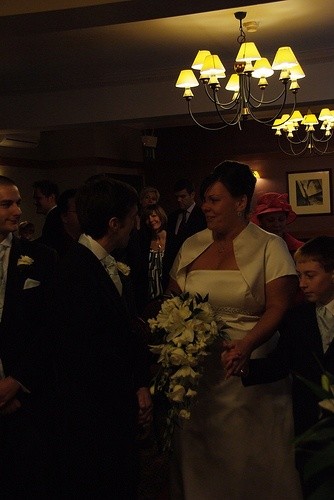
[241,370,245,374]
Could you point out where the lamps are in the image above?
[272,104,334,156]
[176,11,306,131]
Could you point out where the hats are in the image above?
[252,192,297,227]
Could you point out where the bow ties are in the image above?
[105,257,131,277]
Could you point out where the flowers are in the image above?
[17,254,33,267]
[114,261,131,276]
[146,289,231,454]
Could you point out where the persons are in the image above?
[40,174,155,500]
[18,179,208,331]
[162,160,305,500]
[1,175,47,500]
[249,193,304,256]
[221,235,333,500]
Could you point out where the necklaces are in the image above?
[156,240,162,249]
[217,248,226,254]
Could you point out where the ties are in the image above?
[177,210,186,239]
[0,244,7,324]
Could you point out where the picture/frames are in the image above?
[286,168,333,218]
